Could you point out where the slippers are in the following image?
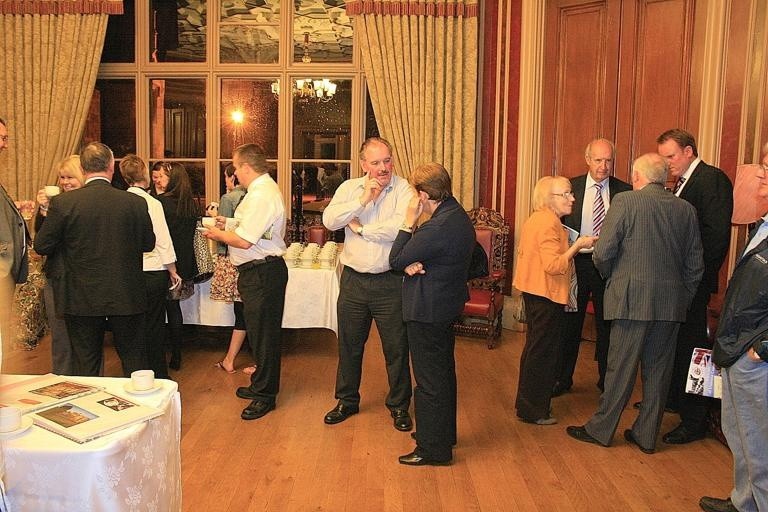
[214,360,236,374]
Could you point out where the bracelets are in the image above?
[401,220,413,230]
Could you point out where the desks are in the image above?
[162,240,341,335]
[0,373,183,512]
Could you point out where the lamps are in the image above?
[270,32,337,105]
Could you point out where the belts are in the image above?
[238,256,282,273]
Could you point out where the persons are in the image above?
[322,136,424,433]
[203,143,289,418]
[556,139,633,400]
[388,161,475,466]
[32,141,200,378]
[290,162,343,242]
[513,175,598,428]
[1,119,31,369]
[563,152,700,453]
[694,140,768,511]
[632,128,721,445]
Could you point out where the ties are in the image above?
[592,183,606,238]
[673,177,685,194]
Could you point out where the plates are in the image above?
[196,228,208,233]
[122,379,164,395]
[0,415,34,437]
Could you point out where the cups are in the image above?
[282,240,340,268]
[131,368,154,390]
[45,186,59,197]
[202,217,216,229]
[20,202,33,221]
[0,407,22,432]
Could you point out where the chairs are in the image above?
[447,208,509,350]
[297,214,336,244]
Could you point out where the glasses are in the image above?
[552,192,575,198]
[0,135,8,141]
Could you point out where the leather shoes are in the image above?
[169,347,181,371]
[391,407,413,431]
[399,452,430,465]
[411,432,457,445]
[699,495,738,512]
[324,399,355,424]
[236,386,260,399]
[241,399,275,420]
[565,418,705,455]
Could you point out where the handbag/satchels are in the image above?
[193,217,215,284]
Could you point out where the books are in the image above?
[685,345,726,400]
[0,370,164,447]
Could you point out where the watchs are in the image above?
[751,345,763,361]
[357,223,365,237]
